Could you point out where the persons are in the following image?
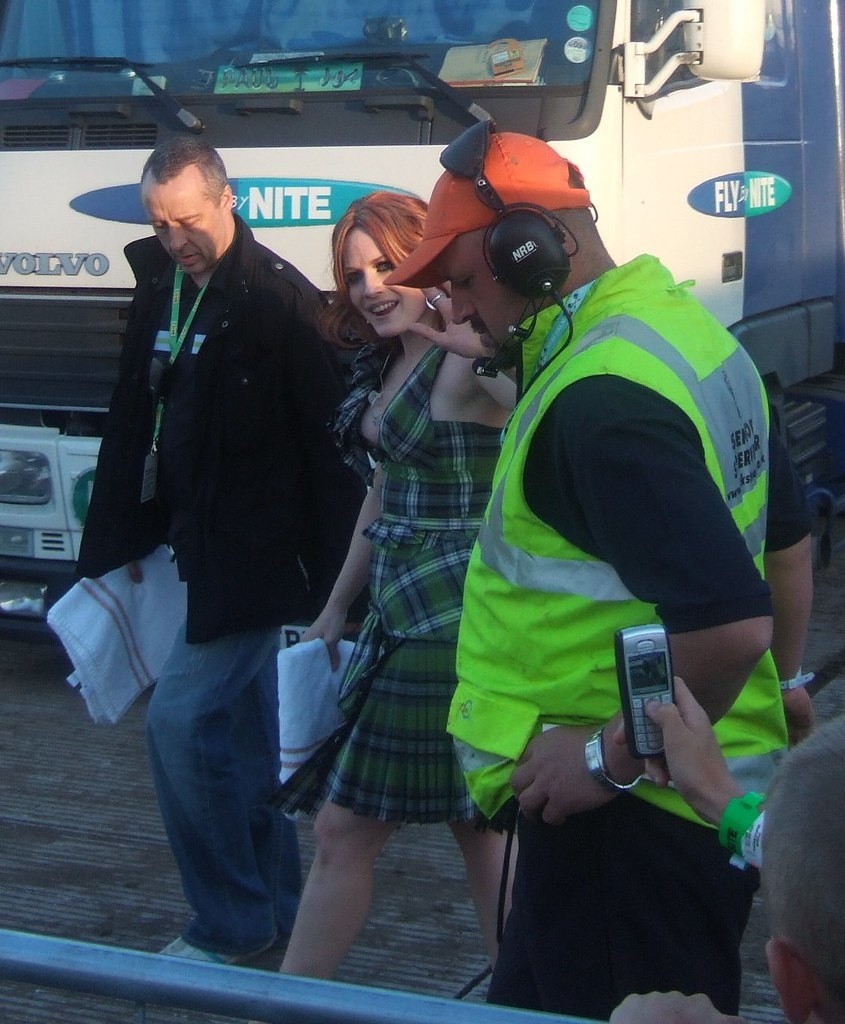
[383,121,822,1023]
[77,129,369,962]
[279,190,518,987]
[609,712,845,1024]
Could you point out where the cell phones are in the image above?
[613,624,675,758]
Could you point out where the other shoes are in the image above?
[160,938,253,970]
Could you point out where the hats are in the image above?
[382,132,591,290]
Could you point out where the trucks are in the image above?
[0,1,845,653]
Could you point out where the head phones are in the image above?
[437,119,570,298]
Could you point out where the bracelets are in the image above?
[719,791,767,860]
[781,668,815,691]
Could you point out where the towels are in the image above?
[277,632,356,823]
[43,542,189,727]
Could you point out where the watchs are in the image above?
[584,723,642,790]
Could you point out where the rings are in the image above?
[430,291,444,306]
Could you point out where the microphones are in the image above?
[472,295,547,375]
